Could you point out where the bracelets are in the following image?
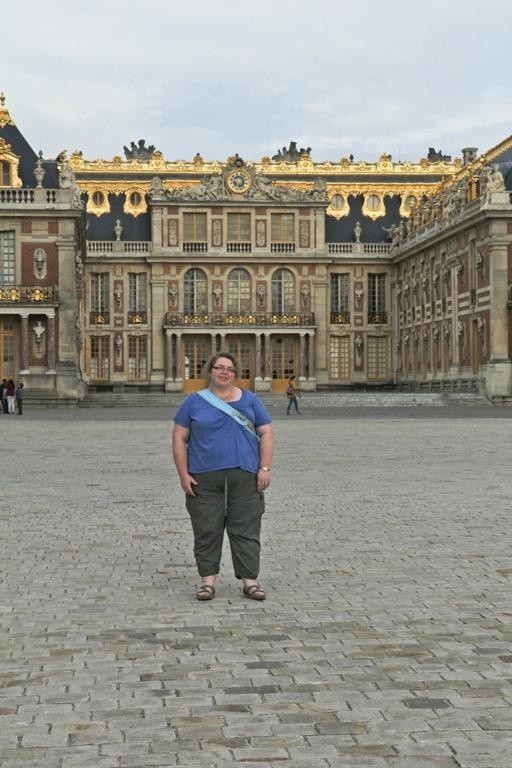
[258,465,270,471]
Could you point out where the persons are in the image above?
[285,374,301,415]
[17,382,24,414]
[173,350,272,601]
[6,379,17,414]
[3,383,7,413]
[1,379,7,412]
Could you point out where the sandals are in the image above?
[196,585,215,600]
[243,584,265,600]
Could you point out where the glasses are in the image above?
[212,366,236,373]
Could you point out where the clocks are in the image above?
[223,167,253,195]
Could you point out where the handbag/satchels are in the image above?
[286,387,294,395]
